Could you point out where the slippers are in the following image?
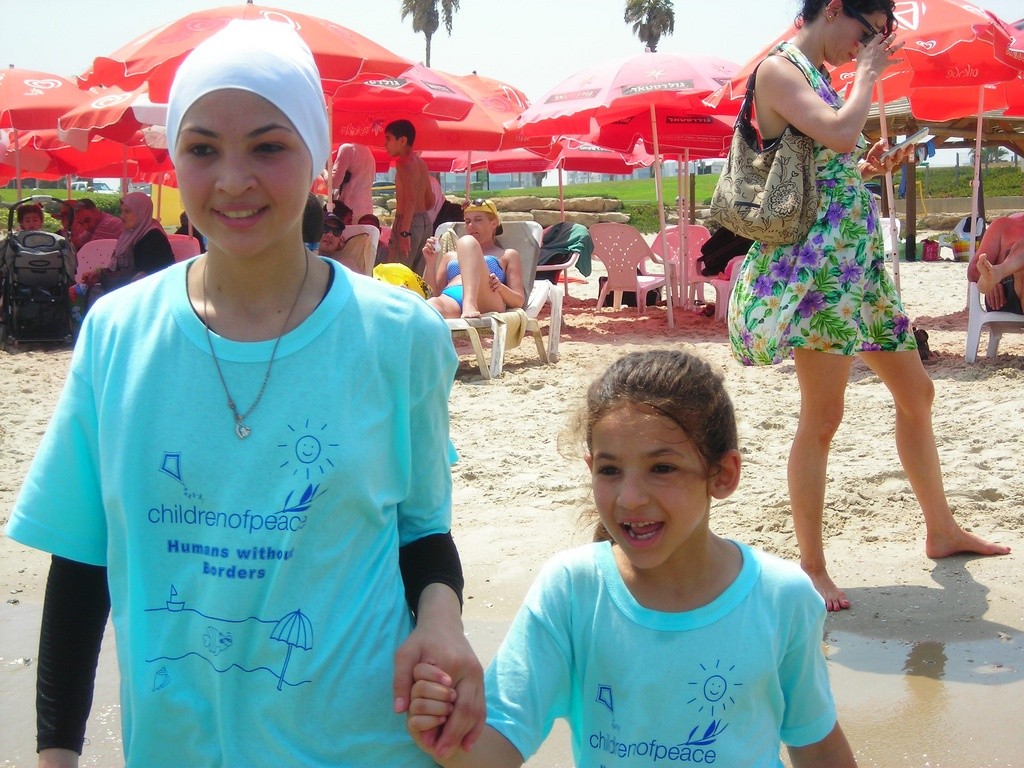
[694,299,707,308]
[701,303,715,316]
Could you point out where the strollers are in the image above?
[0,195,83,346]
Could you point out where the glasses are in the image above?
[842,2,884,47]
[461,197,495,216]
[323,223,343,237]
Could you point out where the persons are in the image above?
[323,200,352,225]
[405,349,858,768]
[3,17,487,768]
[17,205,43,230]
[417,198,526,319]
[968,211,1024,317]
[357,214,389,268]
[84,191,176,295]
[320,142,376,214]
[70,198,124,253]
[380,119,437,277]
[727,0,1011,612]
[318,214,380,278]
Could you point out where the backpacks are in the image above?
[698,226,757,275]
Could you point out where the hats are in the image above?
[463,199,503,236]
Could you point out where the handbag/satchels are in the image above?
[373,262,433,302]
[710,54,819,246]
[599,267,662,307]
[922,240,939,261]
[16,287,74,339]
[952,240,980,262]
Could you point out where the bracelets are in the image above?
[398,231,412,238]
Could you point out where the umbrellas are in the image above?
[0,0,1024,328]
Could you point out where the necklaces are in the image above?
[201,244,307,438]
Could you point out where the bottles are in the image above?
[88,282,104,308]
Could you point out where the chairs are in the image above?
[936,216,1024,364]
[877,217,902,263]
[68,217,746,380]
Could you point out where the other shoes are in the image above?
[913,326,938,364]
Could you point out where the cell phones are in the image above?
[880,127,928,165]
